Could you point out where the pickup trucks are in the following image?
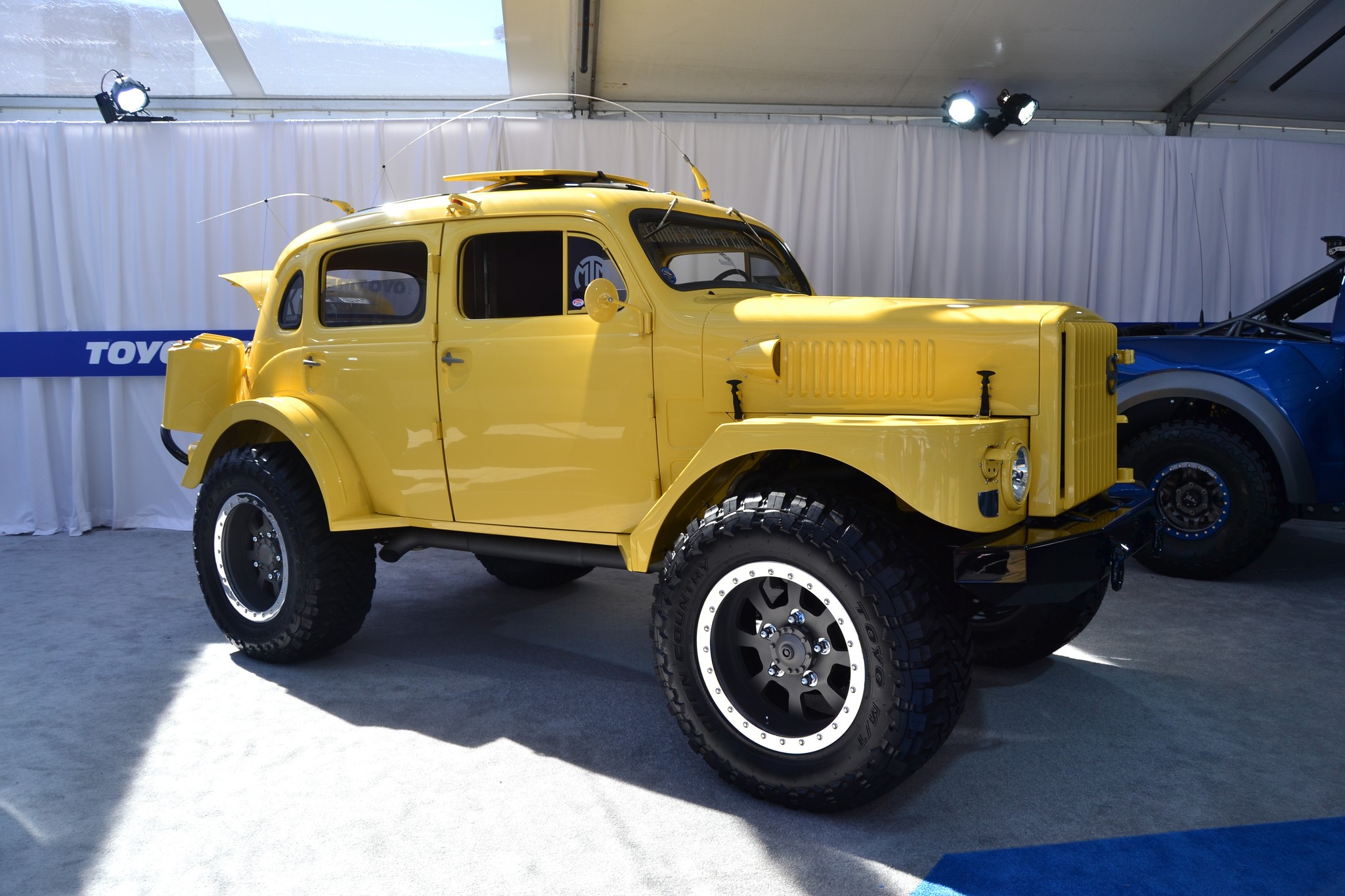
[1116,235,1345,580]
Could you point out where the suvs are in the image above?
[159,144,1158,817]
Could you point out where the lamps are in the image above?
[983,88,1040,139]
[941,89,995,132]
[94,69,177,124]
